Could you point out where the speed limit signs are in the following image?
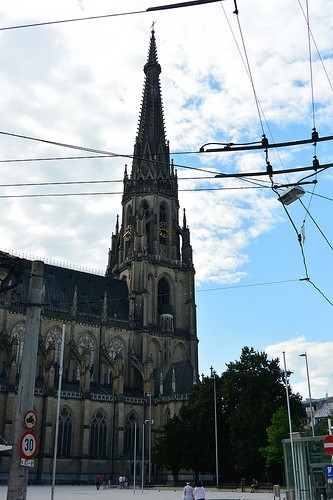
[19,431,38,460]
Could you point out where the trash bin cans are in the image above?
[286,490,295,500]
[273,485,280,500]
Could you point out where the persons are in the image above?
[119,475,128,489]
[96,472,112,490]
[183,481,205,500]
[241,478,258,493]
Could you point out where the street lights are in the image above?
[300,354,315,436]
[208,376,219,489]
[145,393,155,483]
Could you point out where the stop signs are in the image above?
[322,435,333,456]
